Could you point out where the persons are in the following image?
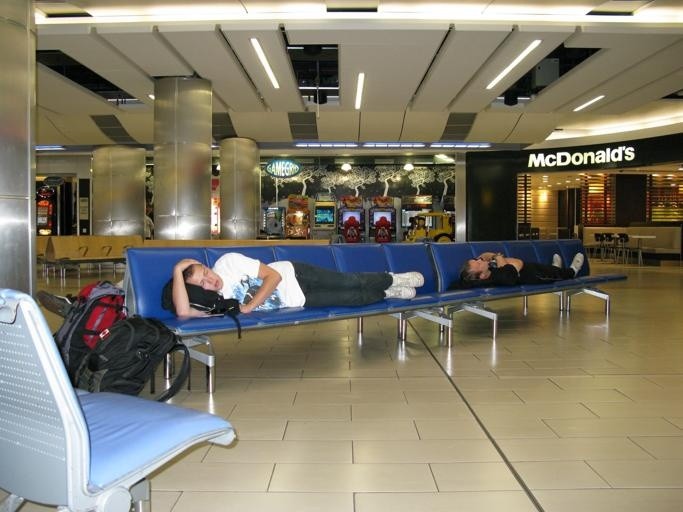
[460,252,584,287]
[172,253,424,317]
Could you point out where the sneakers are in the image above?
[36,290,77,317]
[552,253,562,268]
[384,287,417,300]
[390,271,425,288]
[570,253,584,278]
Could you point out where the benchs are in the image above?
[583,227,682,266]
[126,242,454,394]
[430,237,628,344]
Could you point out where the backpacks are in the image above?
[73,313,191,402]
[54,280,128,377]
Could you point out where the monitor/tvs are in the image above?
[314,201,336,229]
[339,206,366,234]
[370,207,397,232]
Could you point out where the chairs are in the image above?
[36,236,144,279]
[594,232,644,266]
[0,287,236,512]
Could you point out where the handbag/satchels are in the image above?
[162,278,238,320]
